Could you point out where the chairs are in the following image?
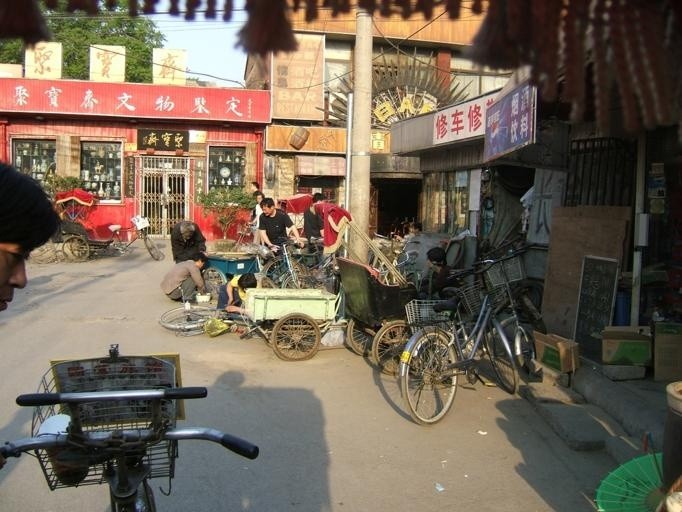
[336,255,420,324]
[60,208,91,234]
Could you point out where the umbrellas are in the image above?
[597,453,682,512]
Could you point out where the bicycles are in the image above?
[399,248,536,427]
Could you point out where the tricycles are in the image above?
[52,187,161,264]
[336,231,546,375]
[158,184,350,363]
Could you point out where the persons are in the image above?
[0,163,62,310]
[411,222,422,236]
[304,193,325,253]
[217,273,257,320]
[422,247,469,311]
[160,221,208,303]
[247,182,304,277]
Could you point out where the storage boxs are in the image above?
[532,330,581,376]
[603,321,682,385]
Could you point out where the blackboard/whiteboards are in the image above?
[573,255,619,366]
